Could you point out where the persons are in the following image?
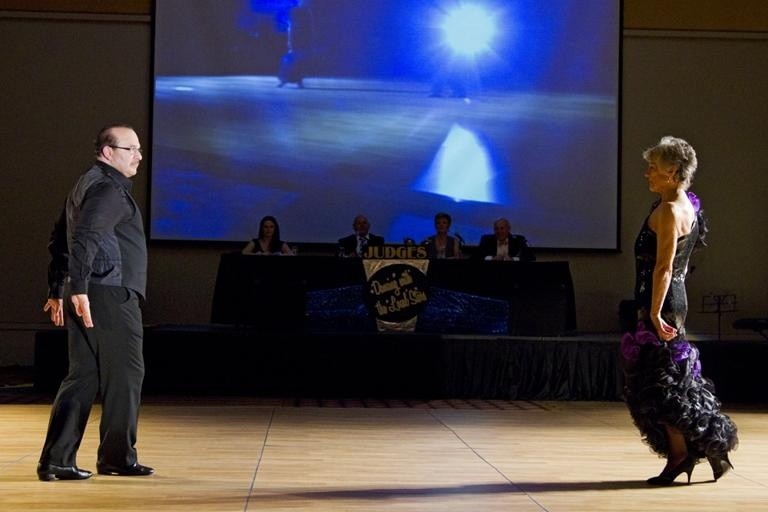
[478,217,528,262]
[420,211,460,259]
[618,136,738,483]
[242,215,295,256]
[336,214,384,258]
[37,125,155,481]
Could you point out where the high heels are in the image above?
[646,452,734,484]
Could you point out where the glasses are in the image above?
[109,145,142,154]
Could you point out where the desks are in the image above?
[217,256,575,337]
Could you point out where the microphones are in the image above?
[455,233,465,244]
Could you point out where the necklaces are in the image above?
[437,236,447,249]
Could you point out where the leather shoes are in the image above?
[96,461,154,476]
[37,461,93,482]
